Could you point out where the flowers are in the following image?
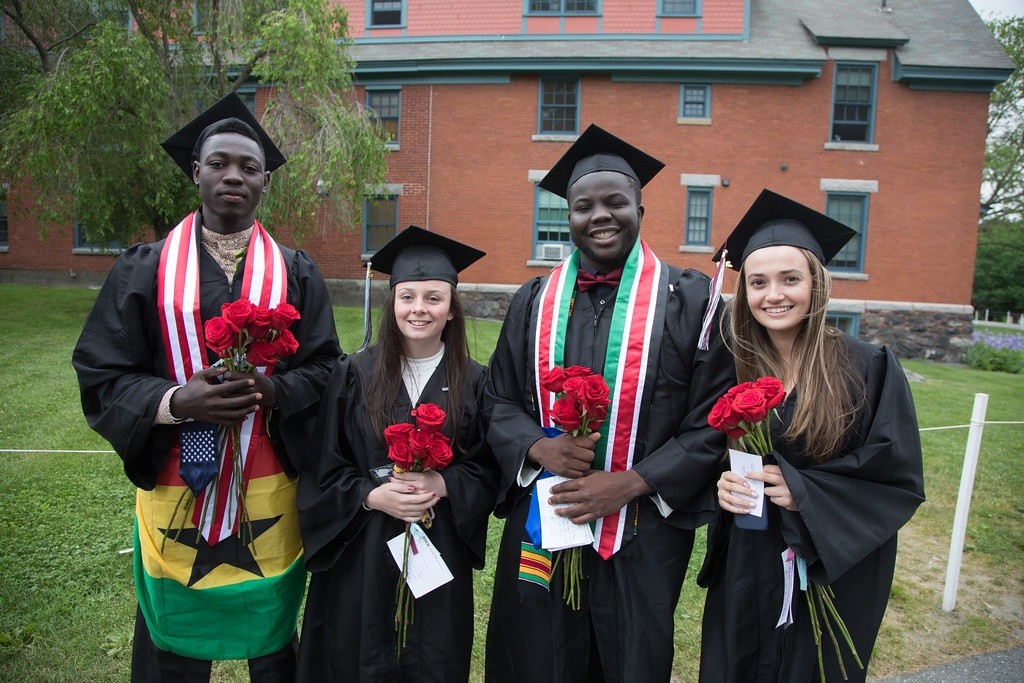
[710,376,868,683]
[367,403,455,660]
[534,361,611,616]
[159,297,301,564]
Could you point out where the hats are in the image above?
[356,225,486,353]
[541,121,666,200]
[697,187,857,351]
[159,92,287,181]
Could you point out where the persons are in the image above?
[476,122,738,683]
[295,225,496,683]
[697,187,926,683]
[72,89,344,683]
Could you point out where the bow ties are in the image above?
[577,268,622,292]
